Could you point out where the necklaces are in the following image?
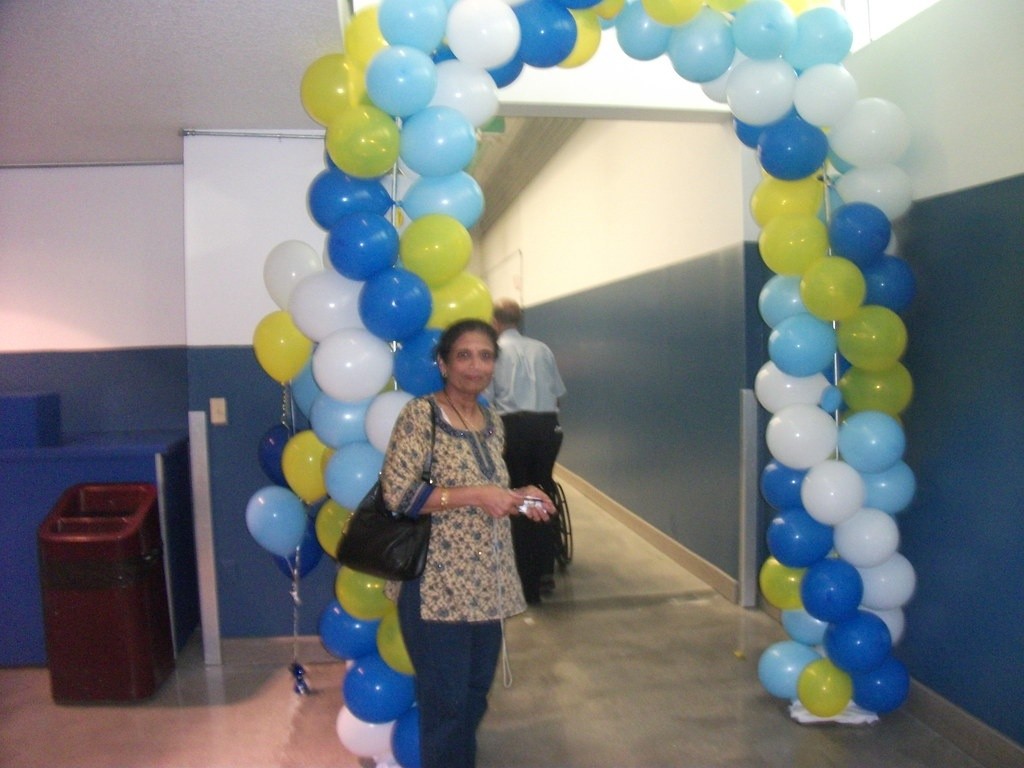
[445,394,478,430]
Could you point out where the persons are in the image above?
[380,316,557,767]
[480,298,567,606]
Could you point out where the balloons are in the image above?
[245,0,918,767]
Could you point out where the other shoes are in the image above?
[540,574,556,593]
[524,582,540,604]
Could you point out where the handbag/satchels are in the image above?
[336,396,435,576]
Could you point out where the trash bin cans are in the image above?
[38,484,167,705]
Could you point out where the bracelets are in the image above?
[440,487,447,511]
